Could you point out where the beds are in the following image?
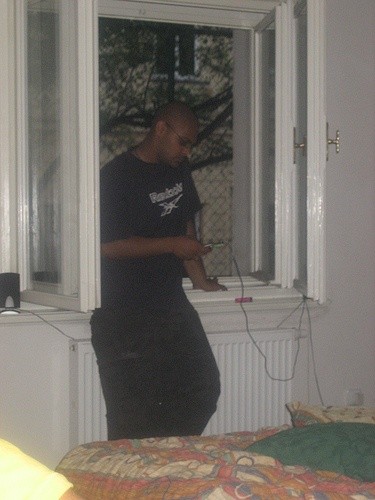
[56,404,375,499]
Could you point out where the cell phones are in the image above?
[203,242,225,248]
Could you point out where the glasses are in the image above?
[168,125,196,151]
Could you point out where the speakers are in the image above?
[0,273,21,315]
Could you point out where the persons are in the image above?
[84,99,229,441]
